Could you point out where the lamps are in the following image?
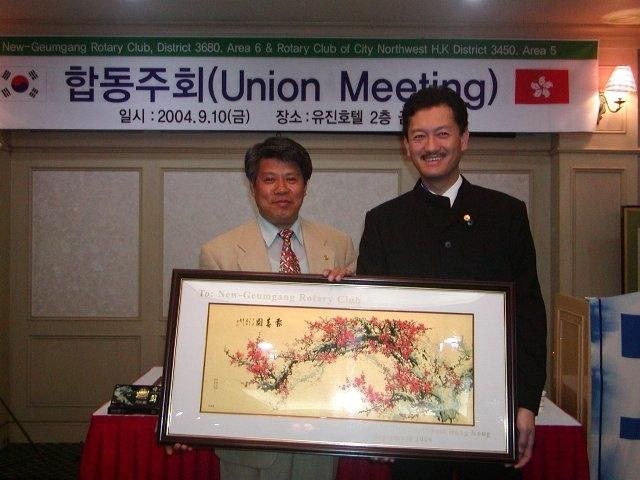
[597,64,638,124]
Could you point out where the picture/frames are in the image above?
[156,269,517,463]
[620,205,640,294]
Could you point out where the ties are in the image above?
[278,229,301,274]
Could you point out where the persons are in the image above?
[195,136,360,479]
[357,85,548,479]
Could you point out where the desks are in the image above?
[82,367,588,479]
